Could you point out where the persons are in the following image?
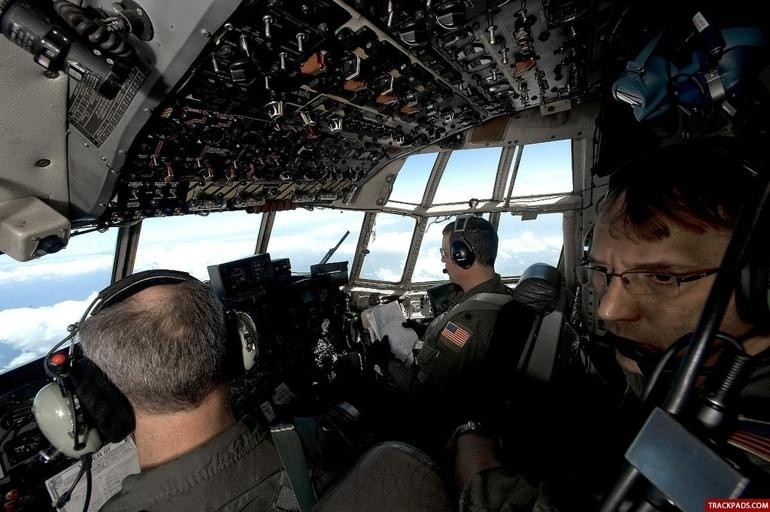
[366,217,513,392]
[79,270,333,512]
[454,141,770,512]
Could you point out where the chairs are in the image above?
[308,262,567,512]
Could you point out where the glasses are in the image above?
[573,260,720,296]
[439,248,449,256]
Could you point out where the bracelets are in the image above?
[454,421,496,443]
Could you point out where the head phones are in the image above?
[451,214,477,270]
[30,265,260,462]
[725,148,770,326]
